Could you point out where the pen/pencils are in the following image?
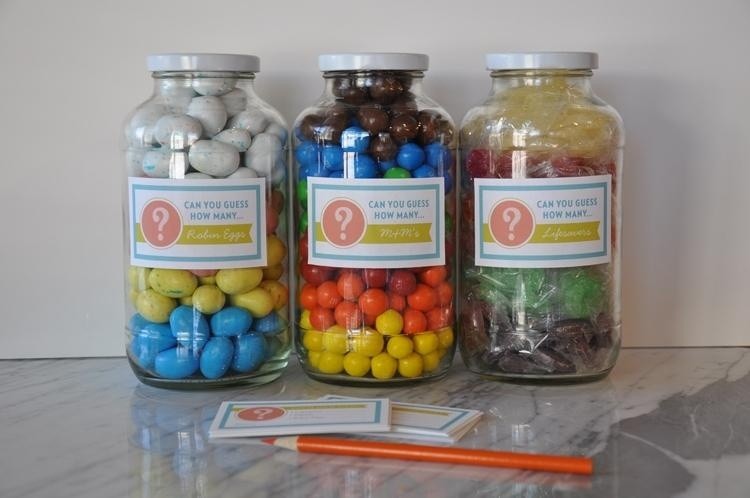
[262,435,592,475]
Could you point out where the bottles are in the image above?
[457,50,627,387]
[291,53,459,391]
[118,53,293,393]
